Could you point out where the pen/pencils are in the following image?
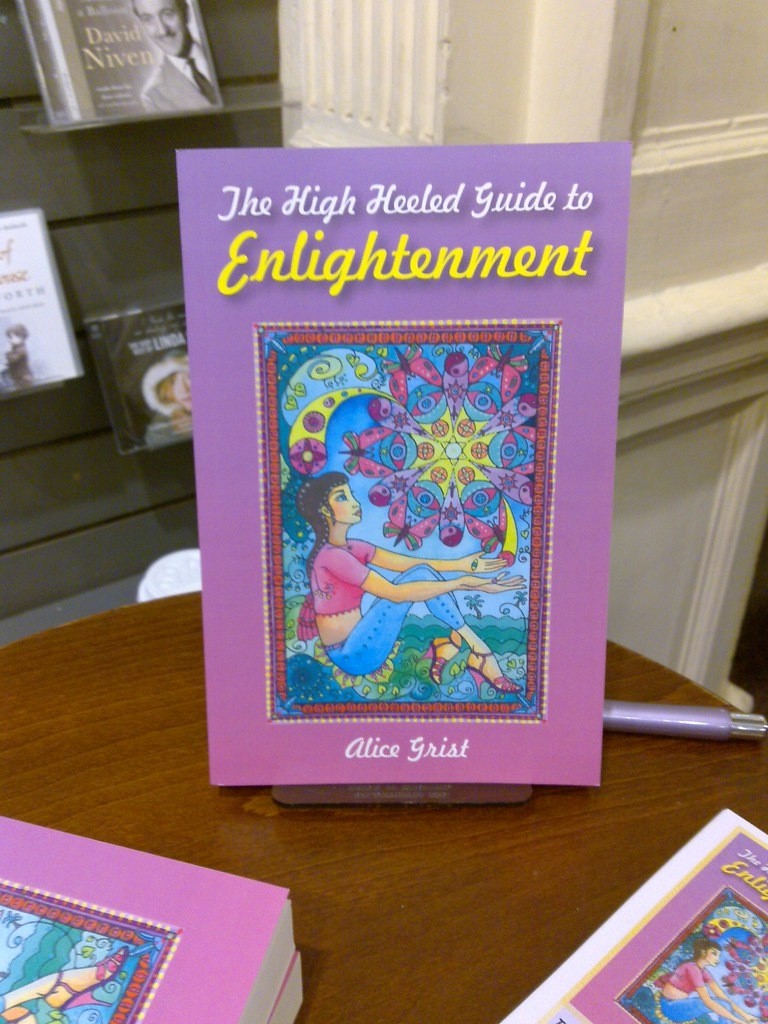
[602,700,768,742]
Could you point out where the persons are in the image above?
[131,0,220,113]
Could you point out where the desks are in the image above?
[1,591,768,1024]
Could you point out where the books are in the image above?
[86,300,194,453]
[15,0,224,127]
[0,816,304,1024]
[175,140,633,802]
[0,207,85,393]
[497,809,768,1024]
